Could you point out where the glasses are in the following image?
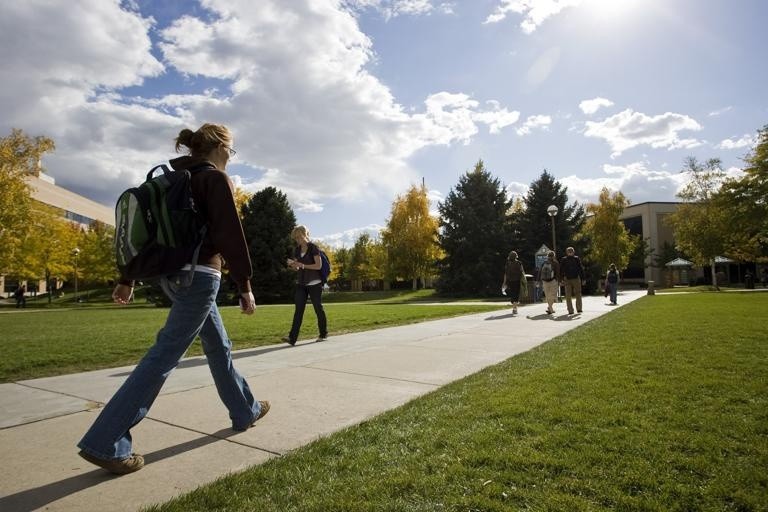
[222,146,236,156]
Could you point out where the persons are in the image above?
[605,263,620,305]
[76,123,272,474]
[537,250,561,314]
[559,247,586,314]
[501,251,528,314]
[281,225,332,345]
[14,285,25,308]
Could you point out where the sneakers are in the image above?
[248,401,270,429]
[316,338,327,342]
[281,337,294,347]
[78,449,145,473]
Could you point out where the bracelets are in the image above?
[302,264,304,270]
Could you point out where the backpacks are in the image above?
[508,263,520,281]
[608,271,617,283]
[540,261,555,282]
[563,259,579,280]
[115,165,200,283]
[319,251,330,280]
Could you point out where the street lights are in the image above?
[545,204,560,300]
[70,247,80,302]
